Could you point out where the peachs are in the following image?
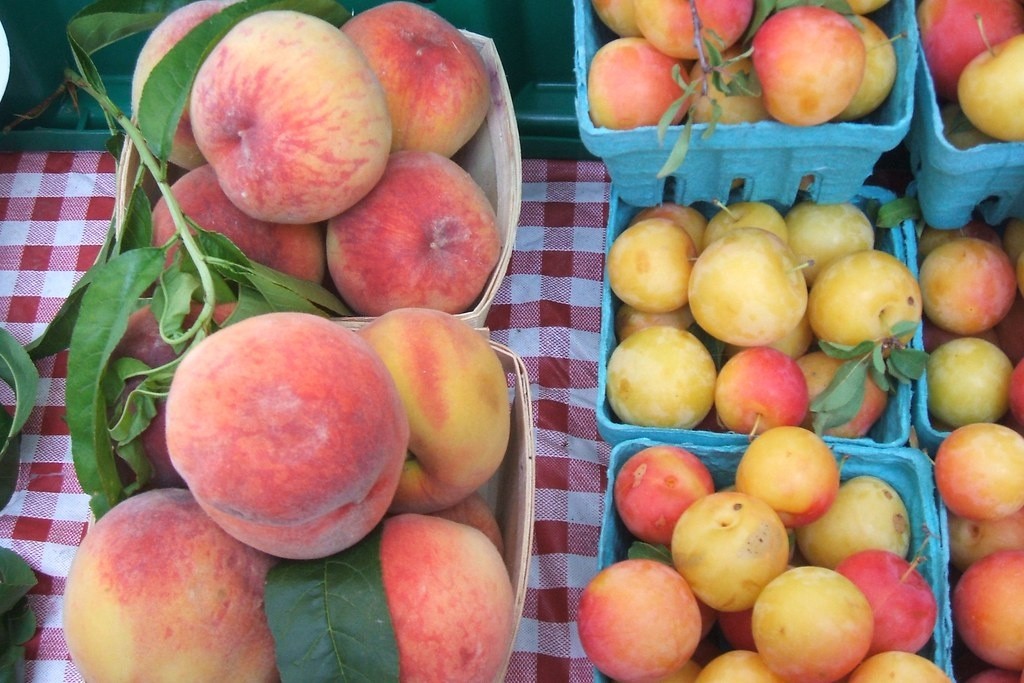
[57,0,518,683]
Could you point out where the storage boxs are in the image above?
[594,170,915,448]
[85,328,538,682]
[590,437,959,683]
[901,175,1024,453]
[114,29,524,328]
[570,0,920,208]
[905,38,1024,230]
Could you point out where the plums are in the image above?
[577,1,1024,683]
